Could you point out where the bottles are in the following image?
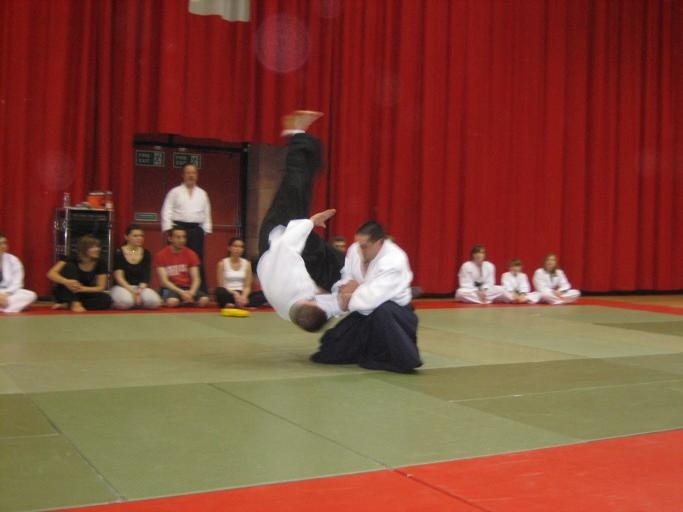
[63,191,71,206]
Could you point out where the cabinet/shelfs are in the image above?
[53,207,113,288]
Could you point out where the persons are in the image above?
[256,111,352,332]
[312,222,422,373]
[160,163,213,295]
[0,235,38,313]
[216,237,267,309]
[46,224,210,312]
[332,237,346,252]
[455,246,581,305]
